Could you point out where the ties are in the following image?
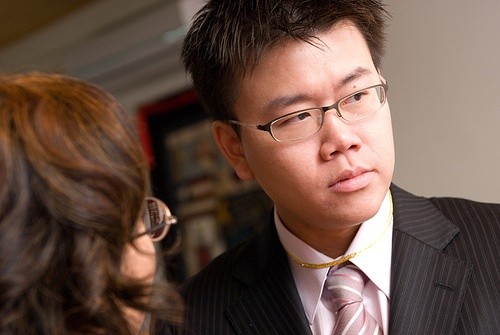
[320,260,385,335]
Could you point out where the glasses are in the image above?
[123,196,177,244]
[225,74,389,143]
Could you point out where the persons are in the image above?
[0,73,182,335]
[150,1,499,335]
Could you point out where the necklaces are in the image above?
[283,189,392,269]
